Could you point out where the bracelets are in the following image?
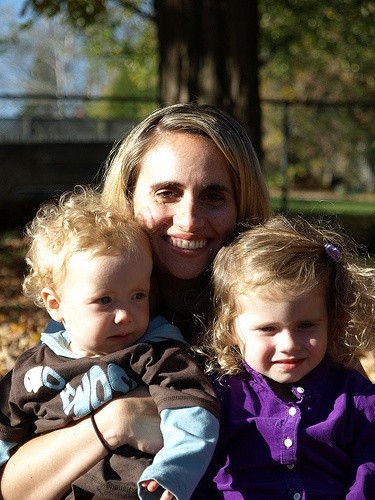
[90,414,117,458]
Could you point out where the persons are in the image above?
[1,103,374,500]
[204,217,374,500]
[0,190,219,500]
